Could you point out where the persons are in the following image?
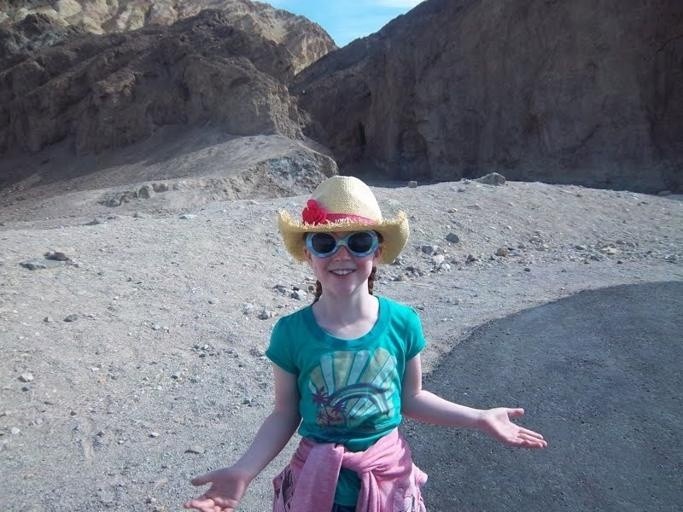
[181,174,549,511]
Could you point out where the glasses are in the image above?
[306,232,379,257]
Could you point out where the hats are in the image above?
[277,174,409,264]
